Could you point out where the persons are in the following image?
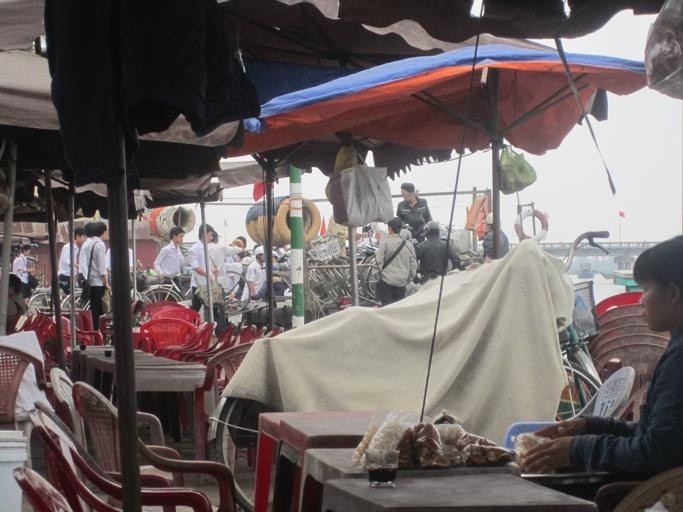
[153,223,291,336]
[376,217,461,307]
[10,245,32,287]
[521,233,682,480]
[59,221,134,332]
[483,212,509,264]
[394,182,432,224]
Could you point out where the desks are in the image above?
[297,446,523,512]
[253,412,397,510]
[315,469,597,511]
[274,414,431,508]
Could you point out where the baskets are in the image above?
[314,238,341,263]
[137,277,150,292]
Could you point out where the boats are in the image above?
[576,263,594,279]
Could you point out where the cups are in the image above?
[79,344,85,350]
[104,350,112,357]
[364,448,399,488]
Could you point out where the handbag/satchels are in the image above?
[375,279,381,301]
[646,0,683,100]
[498,145,536,194]
[330,165,394,227]
[81,280,92,299]
[28,274,38,289]
[101,290,112,314]
[326,145,353,204]
[198,282,222,306]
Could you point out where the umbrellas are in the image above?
[215,50,654,261]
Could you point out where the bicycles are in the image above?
[202,393,276,512]
[556,230,611,423]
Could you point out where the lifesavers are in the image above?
[149,206,194,240]
[246,196,321,246]
[515,210,548,241]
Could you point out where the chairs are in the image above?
[504,291,683,512]
[0,294,303,511]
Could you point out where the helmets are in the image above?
[486,212,494,225]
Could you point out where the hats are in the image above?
[255,244,291,260]
[229,239,244,248]
[420,221,439,236]
[241,257,252,265]
